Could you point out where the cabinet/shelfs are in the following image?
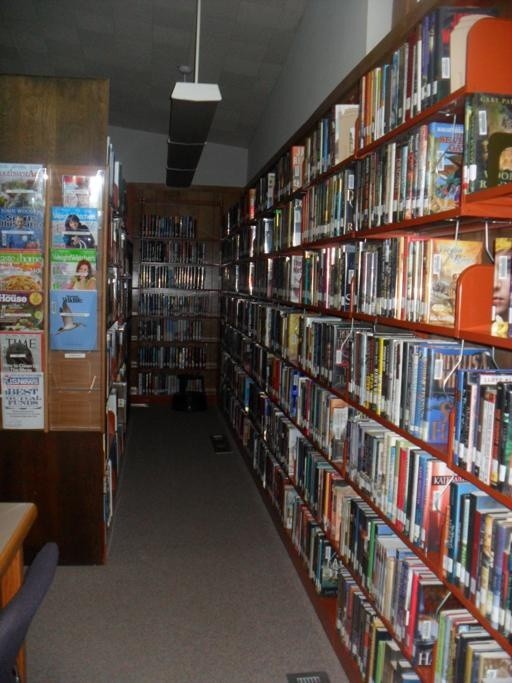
[216,1,511,682]
[124,180,222,406]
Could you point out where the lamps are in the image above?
[164,1,226,190]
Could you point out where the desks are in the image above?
[0,499,40,682]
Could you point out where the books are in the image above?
[0,163,105,432]
[222,292,510,682]
[107,138,131,541]
[221,4,512,339]
[134,212,220,404]
